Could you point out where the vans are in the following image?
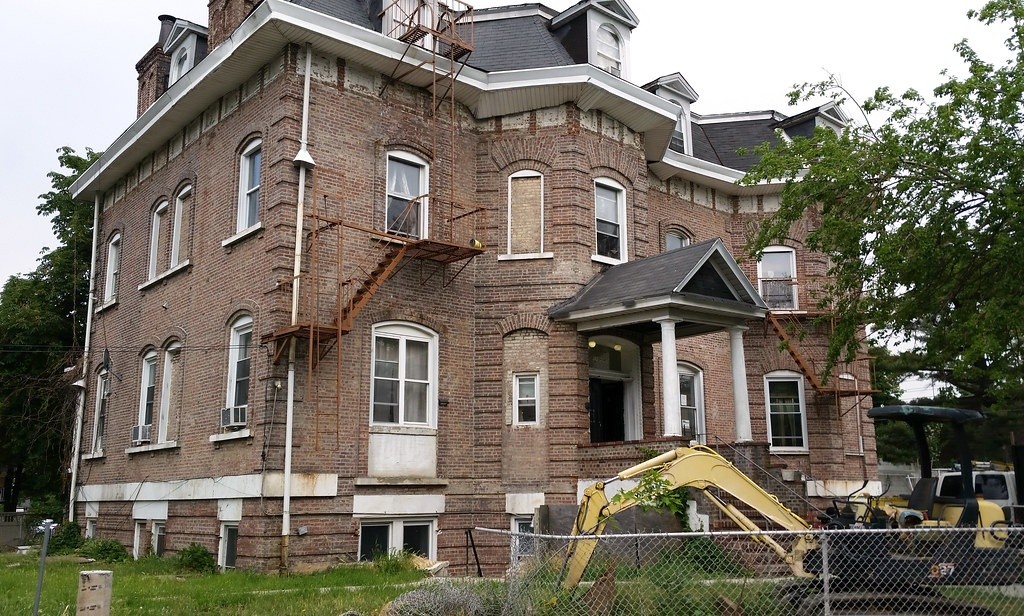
[934,469,1016,523]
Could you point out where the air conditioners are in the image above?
[220,406,247,427]
[132,425,151,442]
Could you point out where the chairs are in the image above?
[907,476,938,519]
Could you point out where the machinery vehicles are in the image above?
[553,385,1024,616]
